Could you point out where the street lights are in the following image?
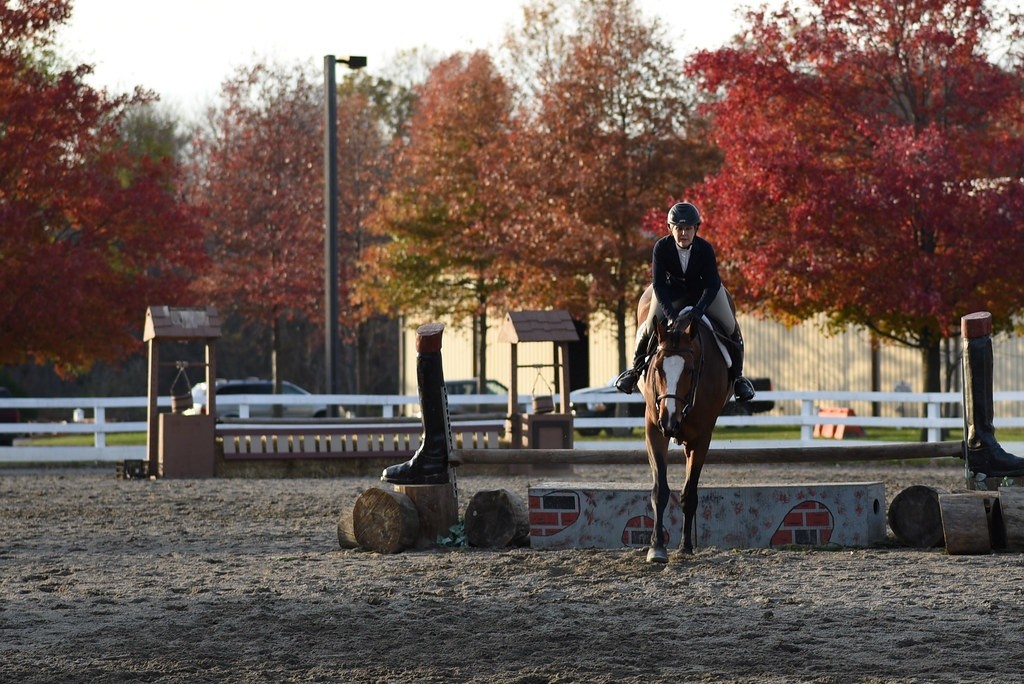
[324,55,368,420]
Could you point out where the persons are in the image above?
[614,201,755,402]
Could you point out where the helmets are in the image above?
[667,202,701,225]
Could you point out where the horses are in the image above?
[643,310,732,566]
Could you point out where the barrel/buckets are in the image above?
[532,372,554,414]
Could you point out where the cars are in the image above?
[190,376,346,418]
[569,366,777,437]
[444,380,508,413]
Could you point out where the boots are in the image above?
[616,333,658,394]
[963,335,1024,475]
[380,353,453,483]
[724,318,754,402]
